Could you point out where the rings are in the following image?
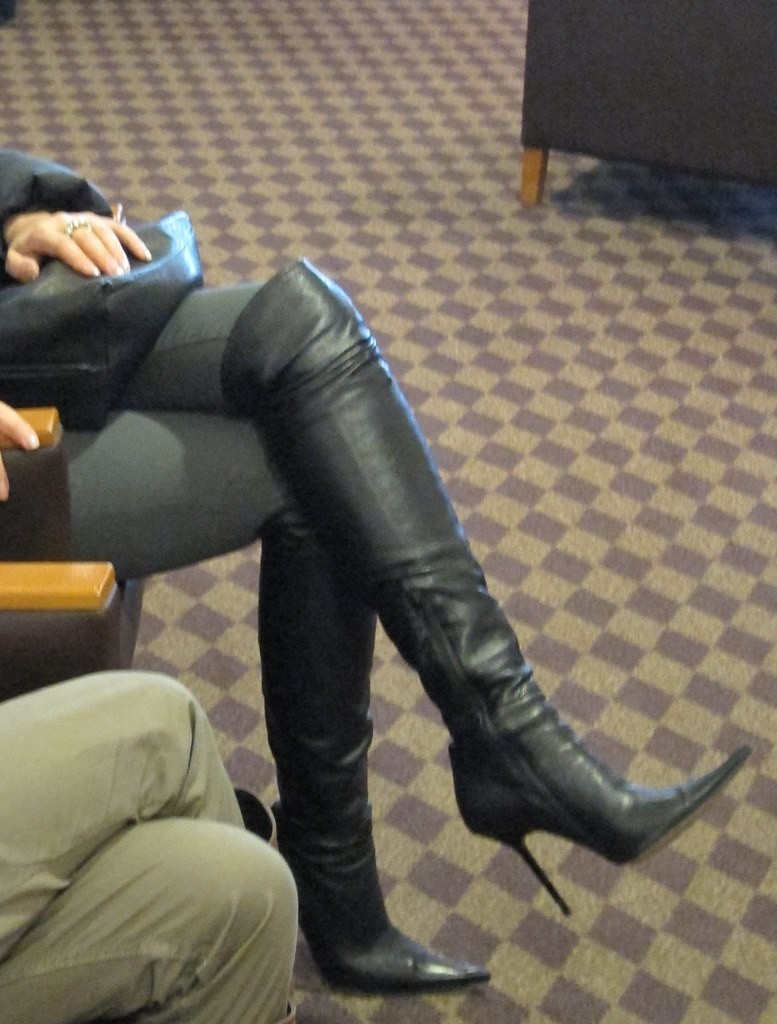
[65,219,92,236]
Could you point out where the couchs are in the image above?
[522,3,775,221]
[0,409,147,692]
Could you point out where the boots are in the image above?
[218,261,751,917]
[257,465,492,994]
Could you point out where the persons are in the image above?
[0,145,754,994]
[1,670,298,1024]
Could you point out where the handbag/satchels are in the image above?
[0,202,200,426]
